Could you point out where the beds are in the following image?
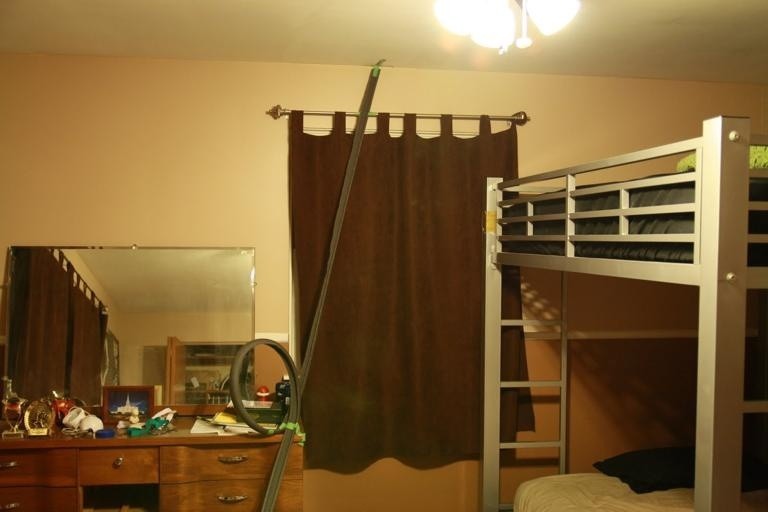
[480,112,766,512]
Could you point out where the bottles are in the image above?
[274,374,291,413]
[255,386,270,401]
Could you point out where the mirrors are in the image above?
[8,245,260,419]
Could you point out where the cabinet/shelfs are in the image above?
[1,416,307,511]
[163,333,255,411]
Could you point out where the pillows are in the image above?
[590,444,765,495]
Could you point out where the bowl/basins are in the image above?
[79,413,104,432]
[62,408,86,430]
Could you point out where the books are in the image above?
[190,412,278,436]
